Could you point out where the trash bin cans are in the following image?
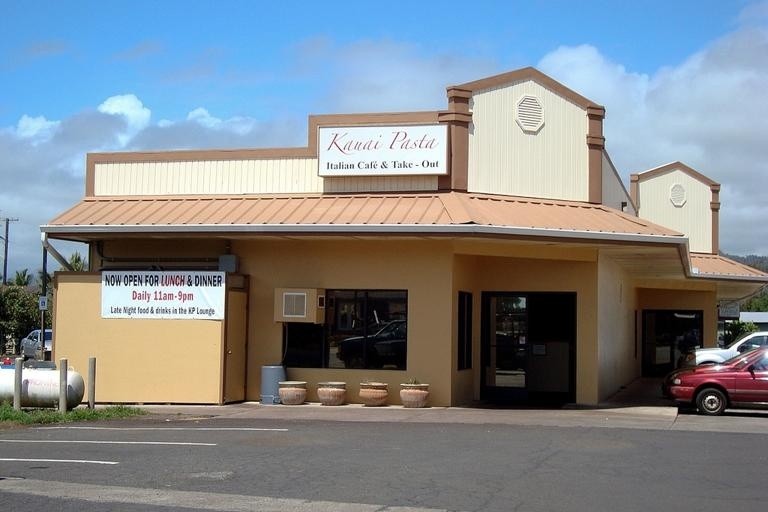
[260,364,287,405]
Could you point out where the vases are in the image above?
[275,380,429,408]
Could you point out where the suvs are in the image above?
[677,330,767,367]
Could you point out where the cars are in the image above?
[21,329,52,359]
[662,345,768,416]
[335,318,406,368]
